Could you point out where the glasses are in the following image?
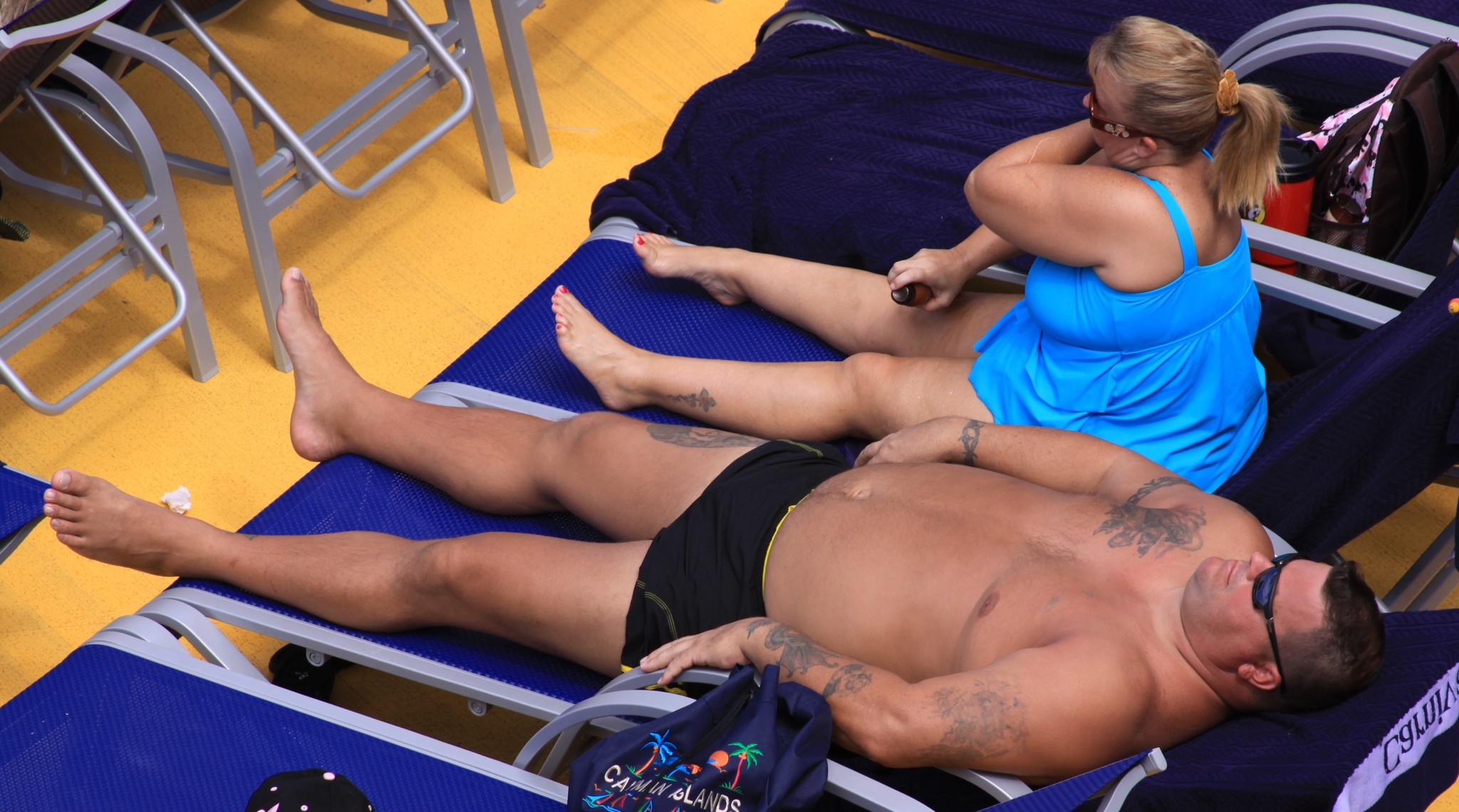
[1089,85,1171,144]
[1251,553,1305,686]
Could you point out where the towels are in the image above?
[587,19,1305,277]
[755,2,1459,120]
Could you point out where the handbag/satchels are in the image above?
[566,662,831,812]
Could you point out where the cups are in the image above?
[1305,193,1362,276]
[1249,137,1319,267]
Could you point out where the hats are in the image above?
[244,769,372,812]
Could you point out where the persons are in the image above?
[552,15,1292,493]
[43,267,1385,776]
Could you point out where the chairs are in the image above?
[0,0,1459,812]
